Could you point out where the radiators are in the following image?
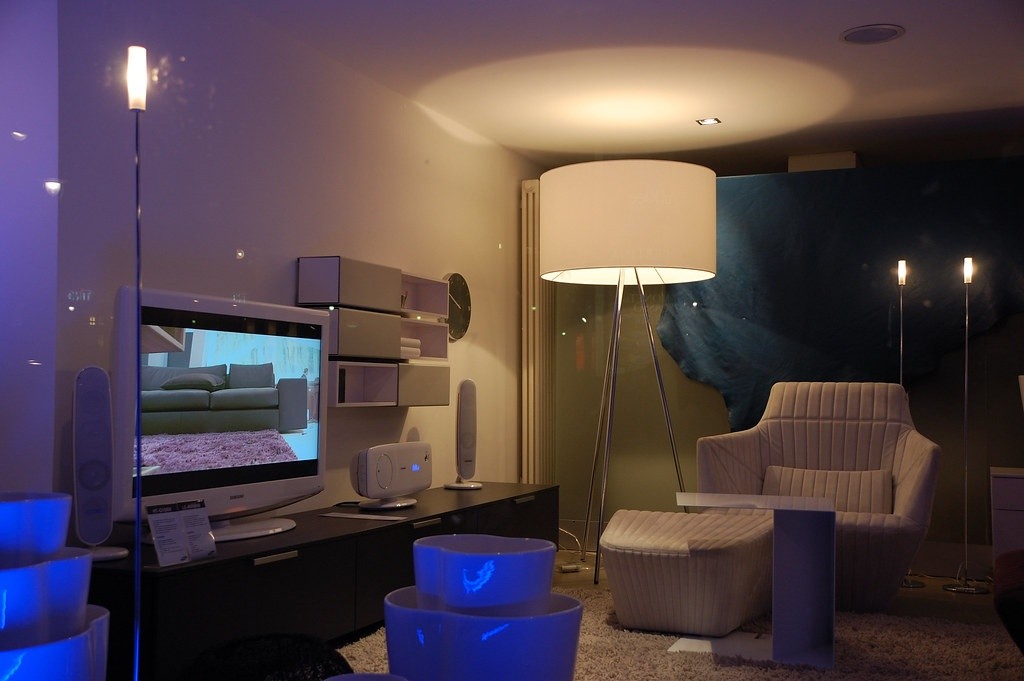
[520,178,556,484]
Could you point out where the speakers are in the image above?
[444,378,482,490]
[70,365,111,547]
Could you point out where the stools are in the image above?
[599,509,774,638]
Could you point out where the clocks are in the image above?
[442,273,471,341]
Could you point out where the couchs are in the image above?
[693,380,942,608]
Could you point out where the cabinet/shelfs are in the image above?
[294,255,451,408]
[85,480,562,681]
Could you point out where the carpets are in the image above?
[336,587,1024,681]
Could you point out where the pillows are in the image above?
[762,464,893,515]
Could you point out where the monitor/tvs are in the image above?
[109,284,331,541]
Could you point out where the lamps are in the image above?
[126,43,150,681]
[541,159,716,587]
[942,256,992,594]
[897,258,926,588]
